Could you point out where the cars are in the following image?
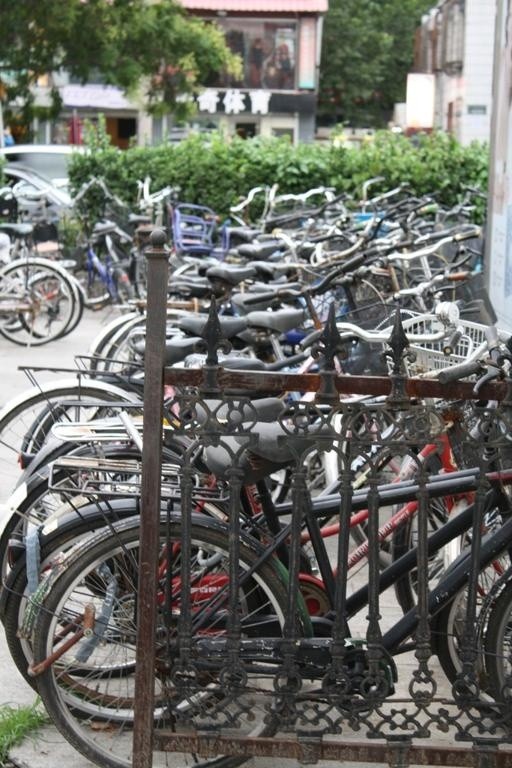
[4,167,76,217]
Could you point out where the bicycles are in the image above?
[0,178,173,346]
[0,176,511,491]
